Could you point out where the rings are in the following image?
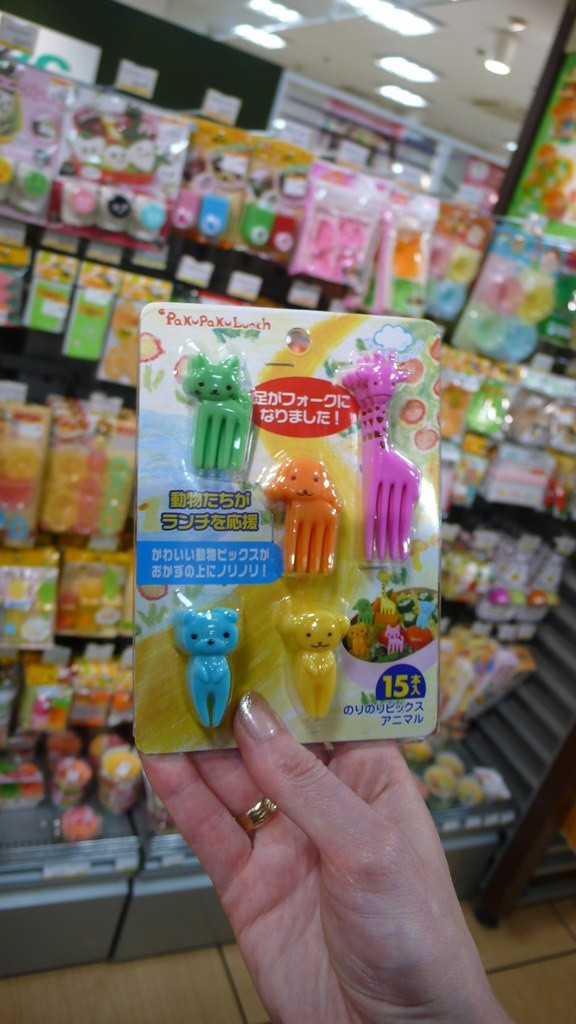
[237,798,277,831]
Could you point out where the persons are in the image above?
[138,690,517,1024]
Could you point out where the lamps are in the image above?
[484,18,528,75]
